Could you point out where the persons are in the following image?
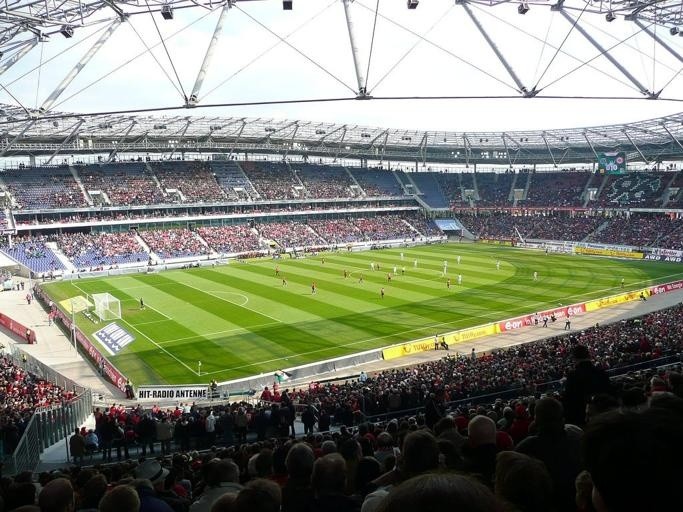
[0,150,270,284]
[442,260,448,276]
[380,286,385,297]
[369,260,373,271]
[138,297,145,311]
[496,260,500,270]
[457,273,462,287]
[375,263,380,270]
[456,255,461,265]
[359,272,365,285]
[620,276,625,288]
[392,266,396,274]
[275,265,279,276]
[533,270,538,281]
[310,280,316,294]
[401,267,406,275]
[321,256,324,264]
[15,278,59,325]
[446,278,450,290]
[385,272,393,281]
[413,258,416,269]
[281,280,288,286]
[343,269,348,279]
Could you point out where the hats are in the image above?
[502,406,513,416]
[454,416,469,427]
[133,458,171,483]
[495,430,512,450]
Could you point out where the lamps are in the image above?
[161,5,173,19]
[408,0,418,8]
[670,27,679,34]
[606,11,616,22]
[283,0,292,10]
[60,24,74,38]
[518,3,529,14]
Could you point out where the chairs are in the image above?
[157,250,198,258]
[89,165,183,191]
[0,164,89,209]
[211,163,250,189]
[444,172,683,259]
[0,242,151,274]
[348,168,411,196]
[295,165,315,180]
